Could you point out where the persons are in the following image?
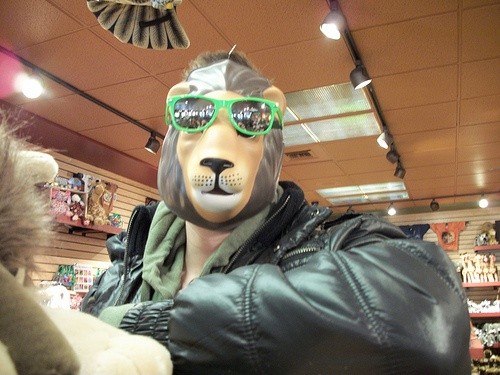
[78,51,471,375]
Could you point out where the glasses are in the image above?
[165,96,283,135]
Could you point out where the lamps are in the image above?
[376,133,393,149]
[145,133,161,154]
[385,152,400,164]
[394,167,406,178]
[24,69,43,99]
[350,68,372,89]
[320,10,345,41]
[430,199,438,211]
[387,203,396,216]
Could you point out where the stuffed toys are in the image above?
[70,185,111,226]
[0,107,174,375]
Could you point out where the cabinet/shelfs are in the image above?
[463,245,500,318]
